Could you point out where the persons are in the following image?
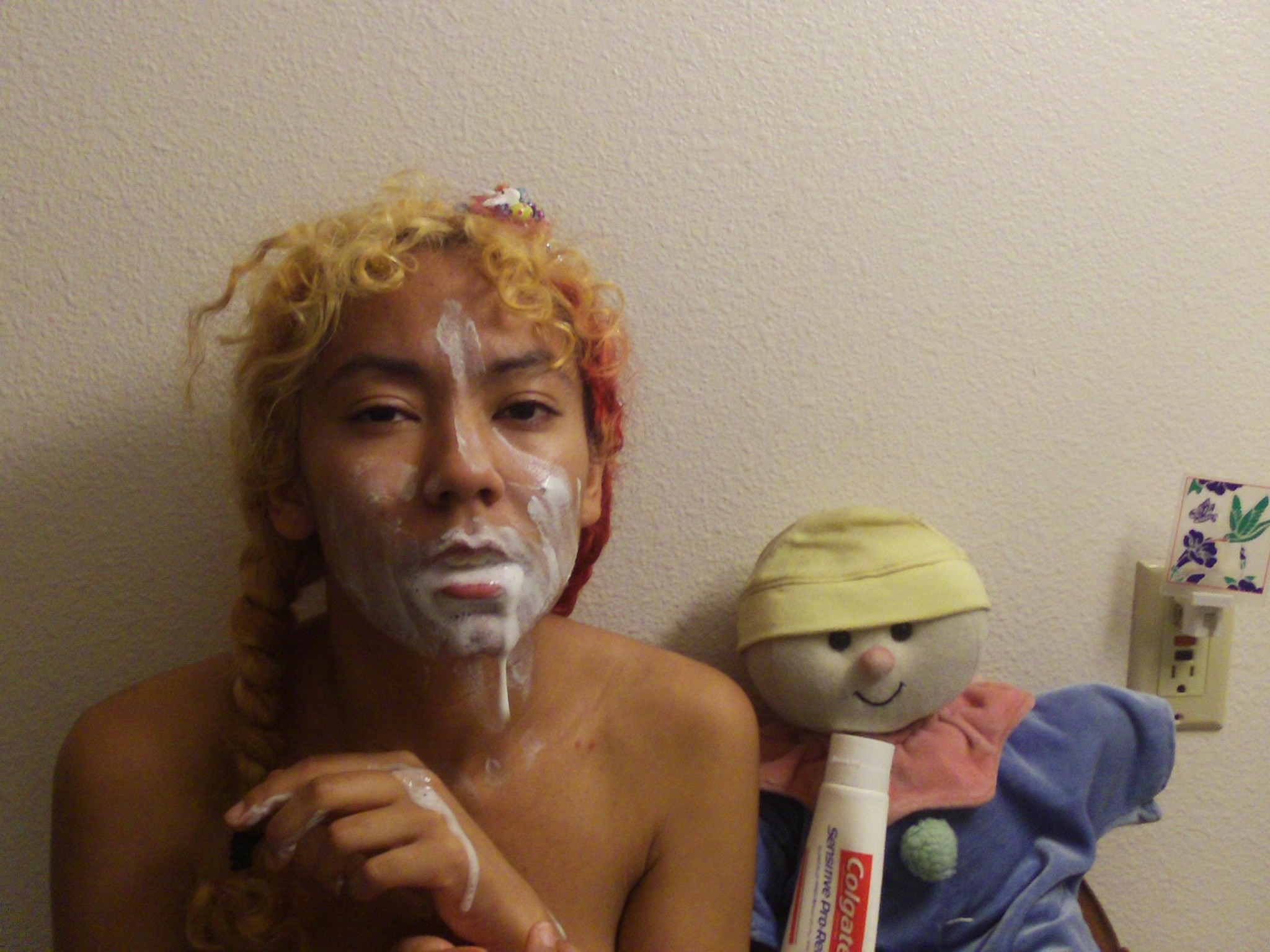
[54,170,761,952]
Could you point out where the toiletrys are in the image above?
[773,731,896,952]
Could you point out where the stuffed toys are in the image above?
[739,512,1174,952]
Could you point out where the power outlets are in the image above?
[1121,558,1236,733]
